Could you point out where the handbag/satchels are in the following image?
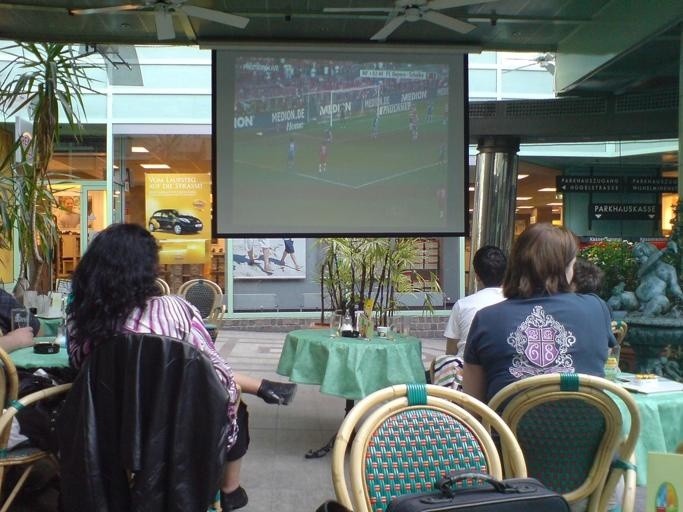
[388,472,571,512]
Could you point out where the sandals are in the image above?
[296,265,306,271]
[249,258,254,264]
[263,265,274,273]
[279,262,287,266]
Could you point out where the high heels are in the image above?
[220,488,248,512]
[256,379,297,406]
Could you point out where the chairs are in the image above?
[178,279,226,345]
[608,320,627,359]
[62,237,81,279]
[397,271,425,292]
[154,278,170,296]
[481,373,640,512]
[0,347,74,512]
[332,384,528,512]
[52,333,229,512]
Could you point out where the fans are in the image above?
[70,0,249,41]
[323,0,488,39]
[509,52,555,74]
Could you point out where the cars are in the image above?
[149,209,203,235]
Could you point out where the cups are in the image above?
[375,326,390,337]
[11,308,29,341]
[329,314,341,338]
[608,344,621,368]
[354,311,365,336]
[342,309,353,332]
[28,290,52,315]
[389,317,400,339]
[399,316,409,337]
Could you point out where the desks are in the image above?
[57,235,80,278]
[395,280,441,292]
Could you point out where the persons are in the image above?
[428,264,606,394]
[436,184,447,218]
[408,106,420,139]
[608,242,683,317]
[0,287,48,358]
[370,115,379,141]
[247,236,274,272]
[281,237,303,270]
[317,141,327,173]
[426,102,434,123]
[287,138,297,168]
[62,224,298,512]
[340,102,349,129]
[465,222,620,443]
[442,102,450,125]
[325,125,333,143]
[442,245,510,360]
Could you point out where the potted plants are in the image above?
[578,240,683,371]
